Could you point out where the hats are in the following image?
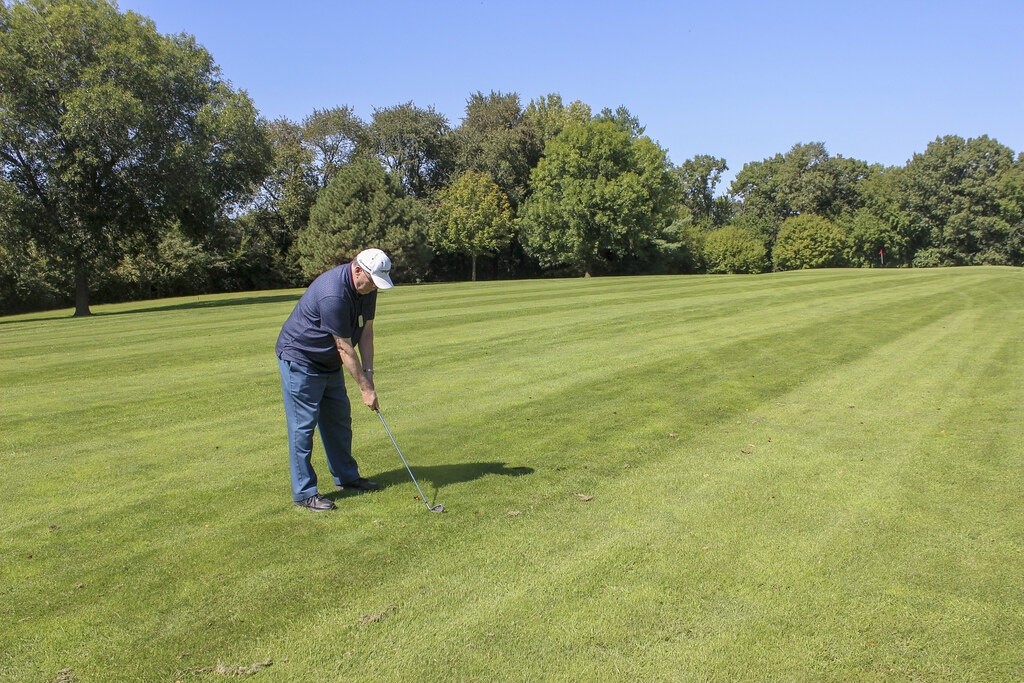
[356,248,393,289]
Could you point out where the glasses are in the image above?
[360,267,377,288]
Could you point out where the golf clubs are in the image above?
[376,409,444,513]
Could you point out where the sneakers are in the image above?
[293,491,337,512]
[336,476,379,491]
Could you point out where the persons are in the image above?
[274,247,394,513]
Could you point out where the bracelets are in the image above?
[362,368,375,374]
